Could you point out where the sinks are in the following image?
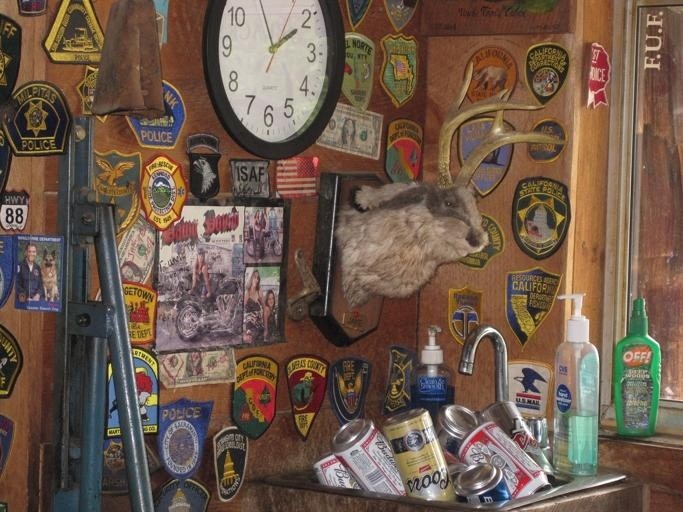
[261,466,631,512]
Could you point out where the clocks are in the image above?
[201,0,347,162]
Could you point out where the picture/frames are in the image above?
[154,197,293,355]
[10,233,63,314]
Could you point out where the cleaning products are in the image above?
[614,298,661,437]
[553,293,599,479]
[410,325,455,415]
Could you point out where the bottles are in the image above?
[478,401,555,483]
[331,418,405,496]
[454,462,510,503]
[433,404,480,452]
[381,409,457,502]
[314,455,360,489]
[455,422,550,500]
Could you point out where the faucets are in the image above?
[458,326,550,466]
